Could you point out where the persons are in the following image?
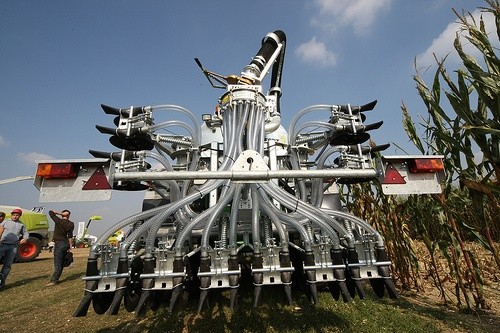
[0,208,29,290]
[48,209,74,286]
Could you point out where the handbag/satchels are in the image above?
[64,251,73,267]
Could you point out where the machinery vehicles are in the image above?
[72,216,102,248]
[0,203,50,264]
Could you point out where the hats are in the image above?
[11,209,23,215]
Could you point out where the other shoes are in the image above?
[44,279,60,287]
